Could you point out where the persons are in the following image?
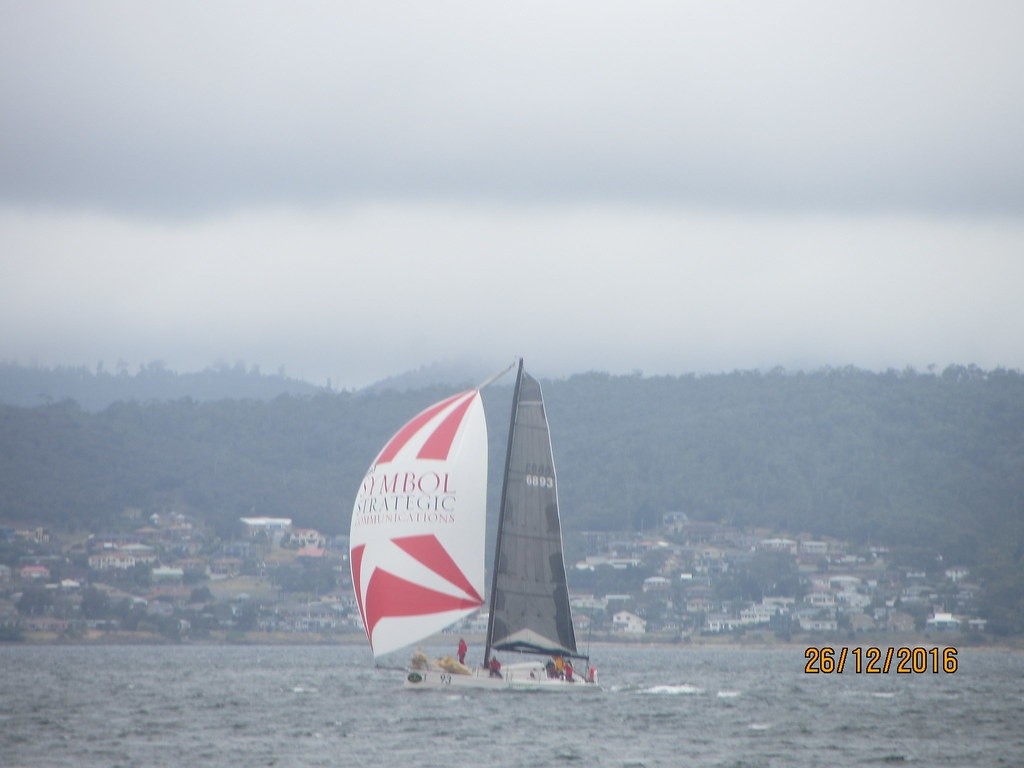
[490,656,501,678]
[547,658,573,683]
[457,639,467,665]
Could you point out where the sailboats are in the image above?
[349,355,604,691]
